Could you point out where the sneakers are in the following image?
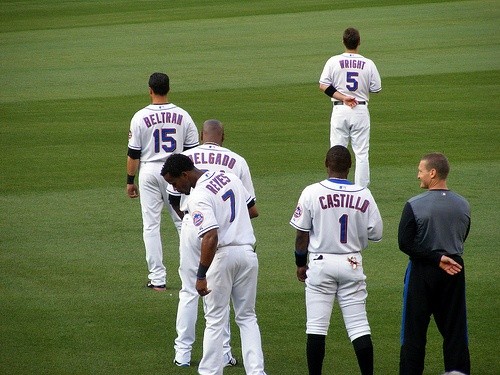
[145,281,166,291]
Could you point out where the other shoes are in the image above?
[173,359,190,367]
[226,357,238,366]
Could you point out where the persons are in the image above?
[164,120,257,368]
[294,145,384,375]
[125,72,201,292]
[318,28,383,188]
[159,153,268,375]
[398,152,472,375]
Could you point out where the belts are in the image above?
[333,101,366,105]
